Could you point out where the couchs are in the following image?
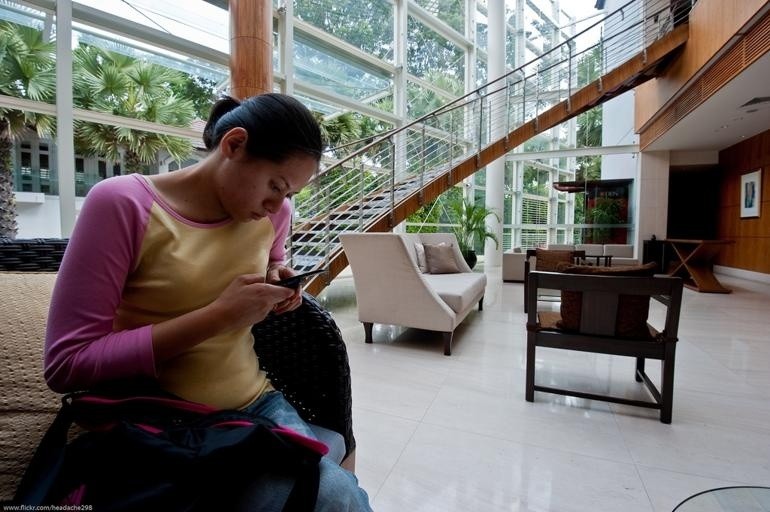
[338,232,487,356]
[502,244,639,282]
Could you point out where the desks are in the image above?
[666,238,736,293]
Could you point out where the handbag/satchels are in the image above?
[13,387,330,512]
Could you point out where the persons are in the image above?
[43,92,374,510]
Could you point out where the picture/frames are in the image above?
[739,166,763,219]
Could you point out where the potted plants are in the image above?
[453,197,500,270]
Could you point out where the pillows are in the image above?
[535,247,571,272]
[422,242,463,274]
[414,242,445,274]
[0,272,94,501]
[556,261,657,341]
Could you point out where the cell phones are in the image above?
[272,269,324,288]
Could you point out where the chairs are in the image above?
[0,237,356,511]
[524,250,586,313]
[525,271,684,424]
